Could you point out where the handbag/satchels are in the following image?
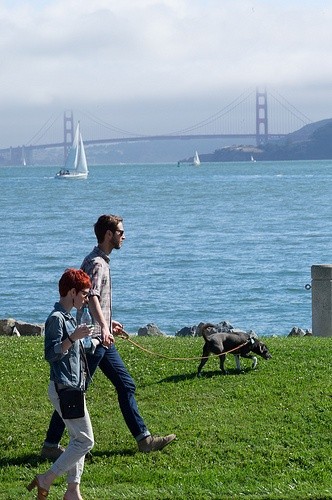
[56,388,85,420]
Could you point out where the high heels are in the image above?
[27,476,48,500]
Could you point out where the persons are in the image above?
[40,214,176,462]
[25,268,115,500]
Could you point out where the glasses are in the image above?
[116,230,124,236]
[81,290,91,298]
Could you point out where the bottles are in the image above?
[81,308,92,348]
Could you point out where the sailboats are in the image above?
[250,155,256,164]
[190,148,200,168]
[54,120,89,181]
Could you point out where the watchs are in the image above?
[67,335,75,344]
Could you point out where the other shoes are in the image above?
[138,433,176,453]
[41,446,65,460]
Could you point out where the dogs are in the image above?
[194,321,273,380]
